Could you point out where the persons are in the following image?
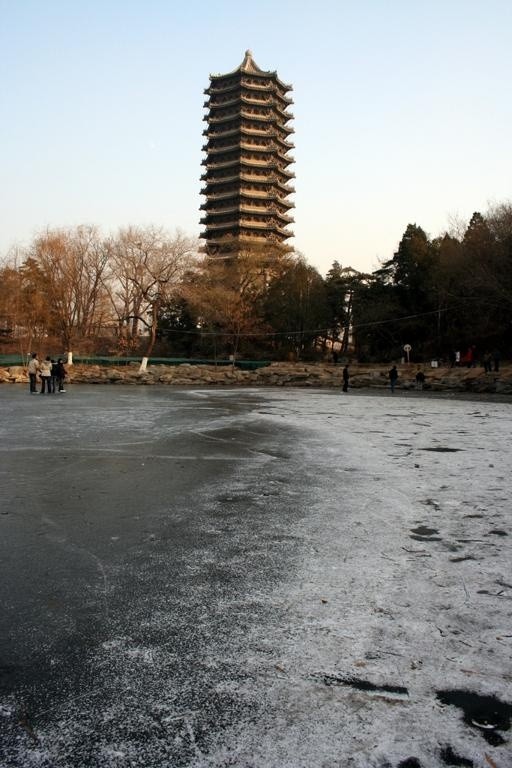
[342,364,350,393]
[447,343,500,374]
[389,364,398,393]
[229,351,235,368]
[412,364,425,391]
[27,352,70,395]
